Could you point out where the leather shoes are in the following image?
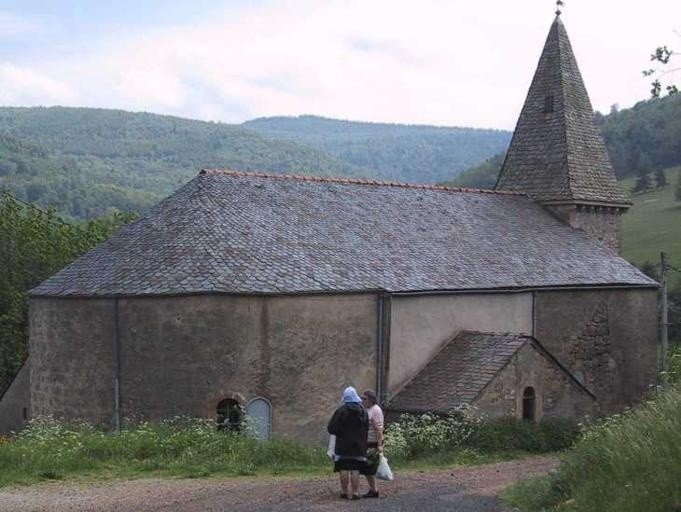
[338,490,378,500]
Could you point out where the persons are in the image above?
[327,385,369,499]
[360,390,385,498]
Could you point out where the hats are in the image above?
[342,387,364,403]
